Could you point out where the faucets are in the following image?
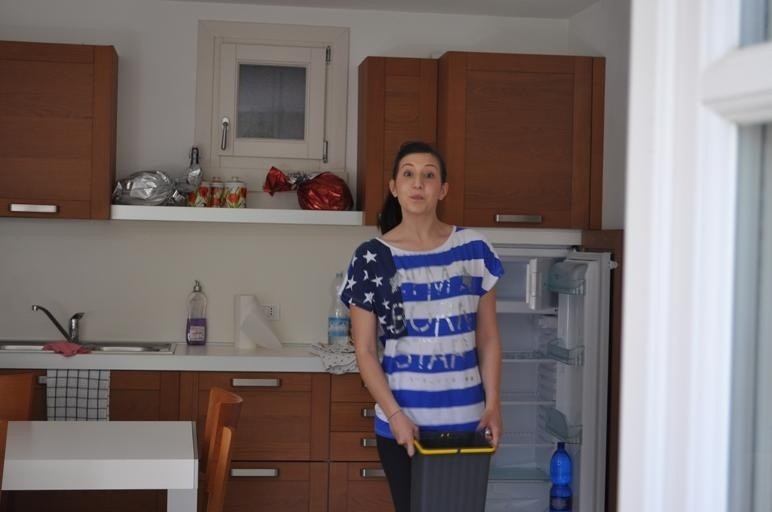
[31,302,82,348]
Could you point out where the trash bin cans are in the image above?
[411,431,493,512]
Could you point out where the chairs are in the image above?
[199,387,243,511]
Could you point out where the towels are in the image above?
[47,370,110,422]
[308,341,358,374]
[43,343,91,357]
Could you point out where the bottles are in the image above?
[224,176,246,208]
[196,176,211,206]
[211,176,225,208]
[550,442,573,511]
[327,272,349,347]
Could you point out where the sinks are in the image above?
[1,342,171,356]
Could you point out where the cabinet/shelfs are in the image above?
[0,38,118,222]
[182,368,330,512]
[331,371,401,512]
[34,366,183,419]
[356,50,605,231]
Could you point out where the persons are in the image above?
[341,140,505,510]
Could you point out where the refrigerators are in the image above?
[484,243,612,512]
